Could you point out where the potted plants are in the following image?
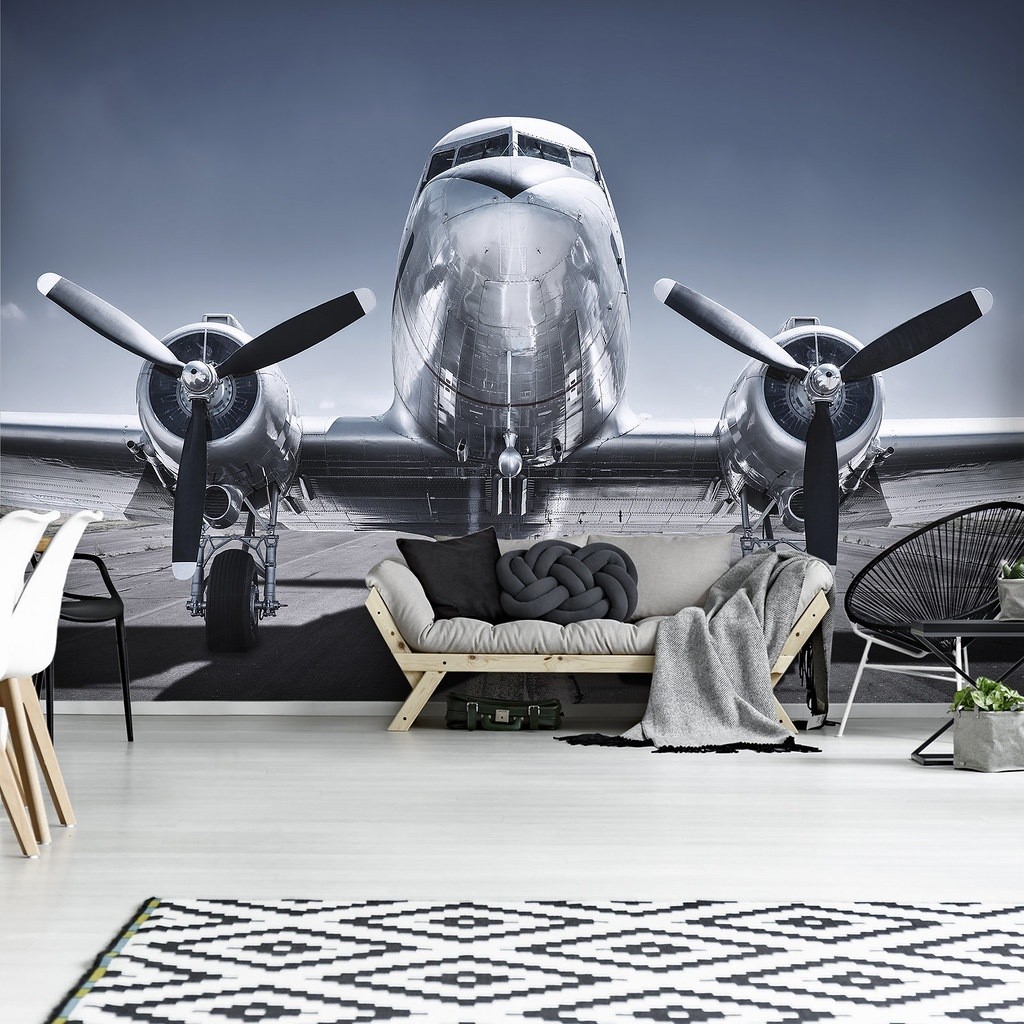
[993,555,1024,622]
[948,675,1024,774]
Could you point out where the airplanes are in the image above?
[0,117,1024,651]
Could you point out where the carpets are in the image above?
[44,896,1024,1023]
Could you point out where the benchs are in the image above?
[365,550,834,732]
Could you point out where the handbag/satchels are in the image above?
[953,688,1024,773]
[444,690,564,731]
[993,560,1024,621]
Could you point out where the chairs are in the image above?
[28,536,135,744]
[834,500,1023,738]
[0,507,108,855]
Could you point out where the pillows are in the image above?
[395,524,510,626]
[583,534,731,623]
